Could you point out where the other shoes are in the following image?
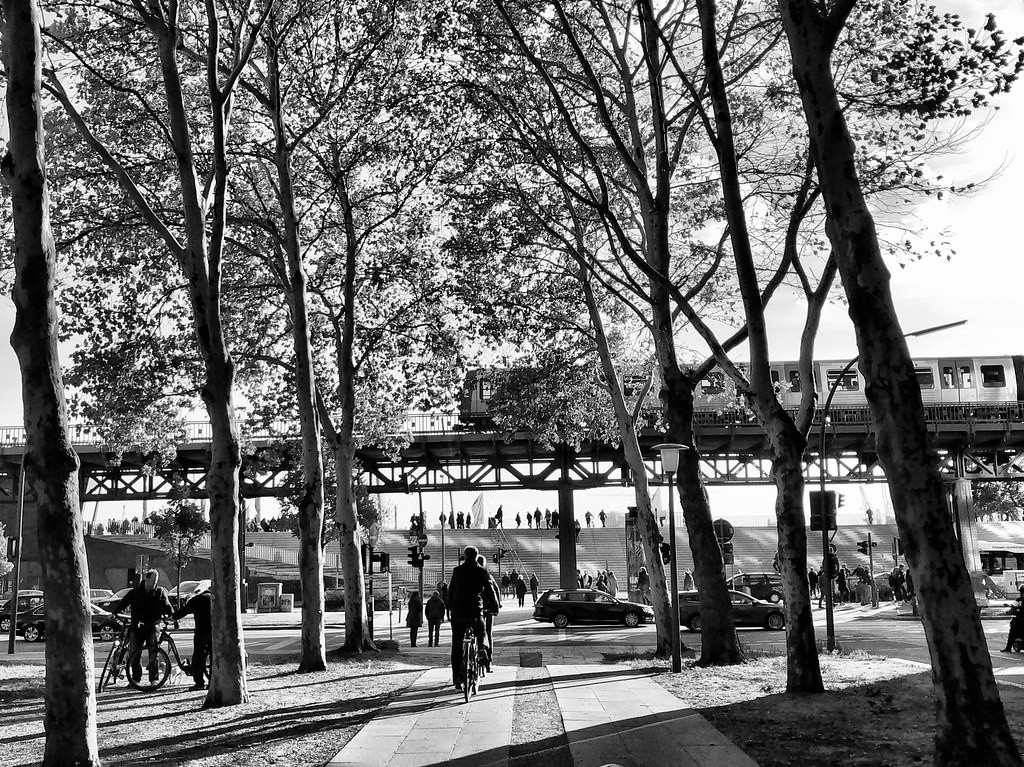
[152,680,160,686]
[478,648,490,663]
[454,682,464,689]
[411,644,418,647]
[435,643,440,647]
[128,679,141,688]
[189,683,204,690]
[428,643,432,647]
[1000,648,1011,653]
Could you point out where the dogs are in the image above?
[158,659,187,685]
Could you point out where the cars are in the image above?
[92,588,133,613]
[676,590,785,632]
[532,589,656,629]
[0,594,44,634]
[166,579,212,611]
[726,572,784,603]
[90,588,116,606]
[16,601,132,643]
[0,590,44,614]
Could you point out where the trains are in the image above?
[458,355,1024,438]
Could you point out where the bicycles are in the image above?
[98,612,133,692]
[478,605,502,677]
[125,615,248,692]
[461,626,483,701]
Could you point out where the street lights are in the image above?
[660,542,672,565]
[648,443,689,675]
[815,320,966,658]
[440,474,445,581]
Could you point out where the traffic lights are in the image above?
[856,541,868,555]
[500,548,508,559]
[493,554,499,563]
[7,537,17,560]
[398,472,411,496]
[407,546,418,568]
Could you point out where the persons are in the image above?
[575,520,582,537]
[502,569,527,608]
[577,569,619,597]
[446,545,501,693]
[495,505,502,528]
[808,566,838,608]
[637,567,651,605]
[516,512,521,528]
[97,523,104,535]
[865,507,873,524]
[425,591,445,648]
[246,516,280,533]
[684,572,694,591]
[530,572,539,607]
[167,590,213,690]
[439,511,472,530]
[406,591,423,647]
[889,564,915,603]
[437,580,451,623]
[112,569,174,687]
[585,510,594,528]
[1000,584,1024,652]
[598,509,608,528]
[122,517,138,535]
[527,507,559,529]
[773,550,779,571]
[836,564,872,605]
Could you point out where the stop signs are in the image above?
[417,534,429,547]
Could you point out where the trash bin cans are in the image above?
[280,594,294,612]
